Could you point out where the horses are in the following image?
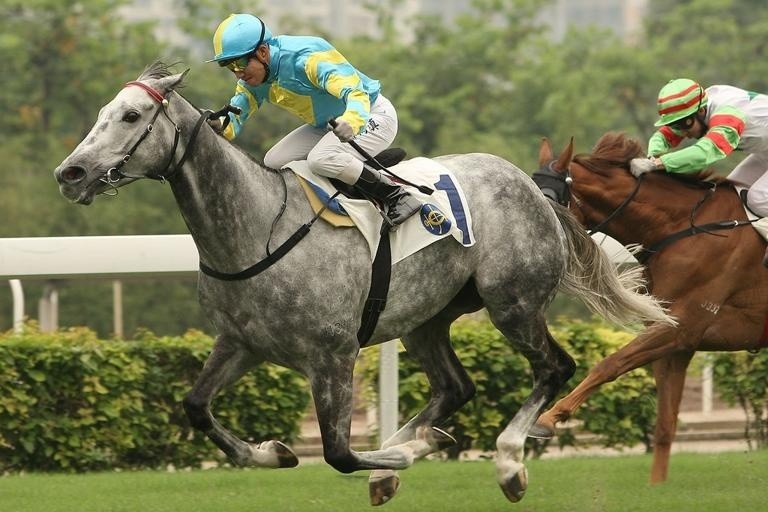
[526,130,768,483]
[52,58,680,508]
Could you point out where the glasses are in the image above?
[218,55,253,72]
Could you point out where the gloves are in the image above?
[630,158,655,178]
[201,108,221,130]
[327,120,353,142]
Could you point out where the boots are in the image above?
[352,163,422,235]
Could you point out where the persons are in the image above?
[199,12,423,236]
[629,78,768,268]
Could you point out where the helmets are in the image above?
[654,78,708,127]
[205,14,272,63]
